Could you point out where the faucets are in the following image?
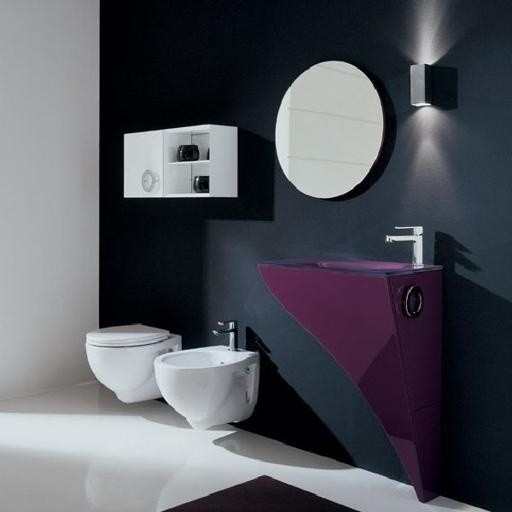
[386,226,425,265]
[212,319,239,350]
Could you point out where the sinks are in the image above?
[154,345,260,428]
[282,260,408,271]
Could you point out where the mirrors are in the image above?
[273,60,386,200]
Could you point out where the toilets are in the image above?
[83,324,181,403]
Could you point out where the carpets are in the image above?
[159,474,362,511]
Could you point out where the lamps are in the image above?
[409,62,433,106]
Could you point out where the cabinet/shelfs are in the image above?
[124,125,237,199]
